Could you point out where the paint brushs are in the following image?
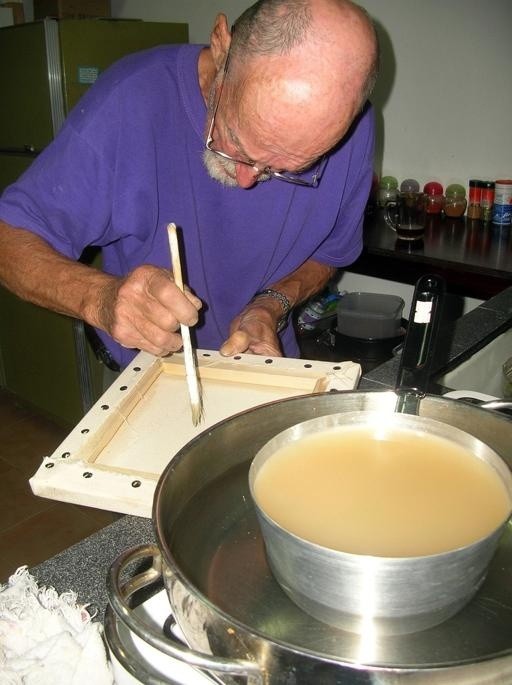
[167,223,202,427]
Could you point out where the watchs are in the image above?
[256,290,290,334]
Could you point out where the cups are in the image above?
[383,192,428,240]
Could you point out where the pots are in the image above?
[106,273,511,683]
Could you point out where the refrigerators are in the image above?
[1,20,189,434]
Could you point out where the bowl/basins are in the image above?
[247,410,511,633]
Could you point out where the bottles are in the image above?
[297,280,338,333]
[366,173,512,226]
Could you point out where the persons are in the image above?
[0,0,381,356]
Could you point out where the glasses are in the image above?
[206,26,325,187]
